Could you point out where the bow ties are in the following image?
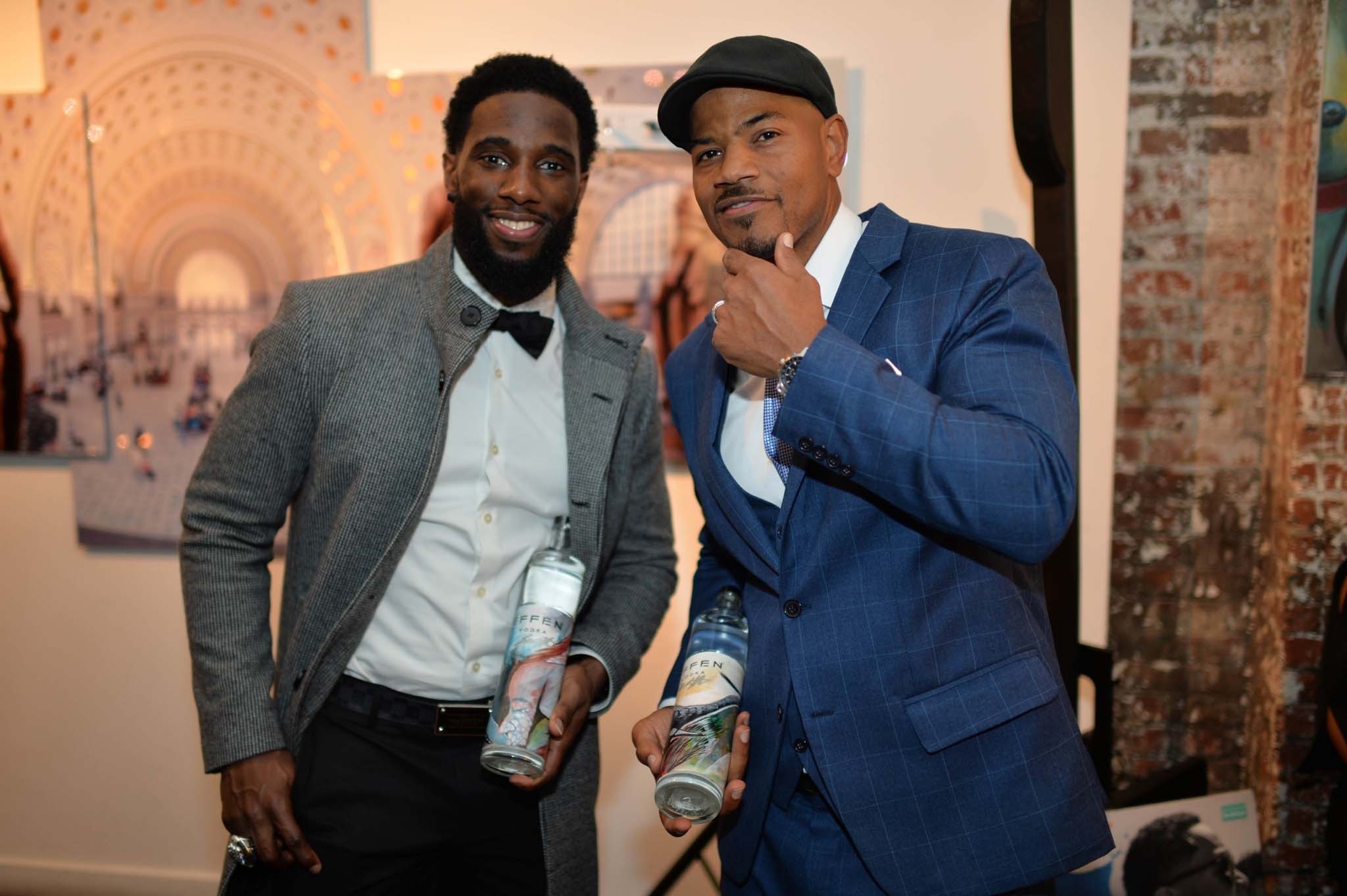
[490,307,554,361]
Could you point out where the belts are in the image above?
[334,679,488,739]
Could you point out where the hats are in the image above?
[656,34,838,150]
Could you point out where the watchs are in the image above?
[774,343,809,399]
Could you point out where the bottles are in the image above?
[655,584,752,822]
[480,514,587,777]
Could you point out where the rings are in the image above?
[711,299,725,324]
[226,832,258,870]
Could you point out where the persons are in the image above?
[1117,809,1251,895]
[1306,555,1347,894]
[188,60,681,896]
[631,40,1122,896]
